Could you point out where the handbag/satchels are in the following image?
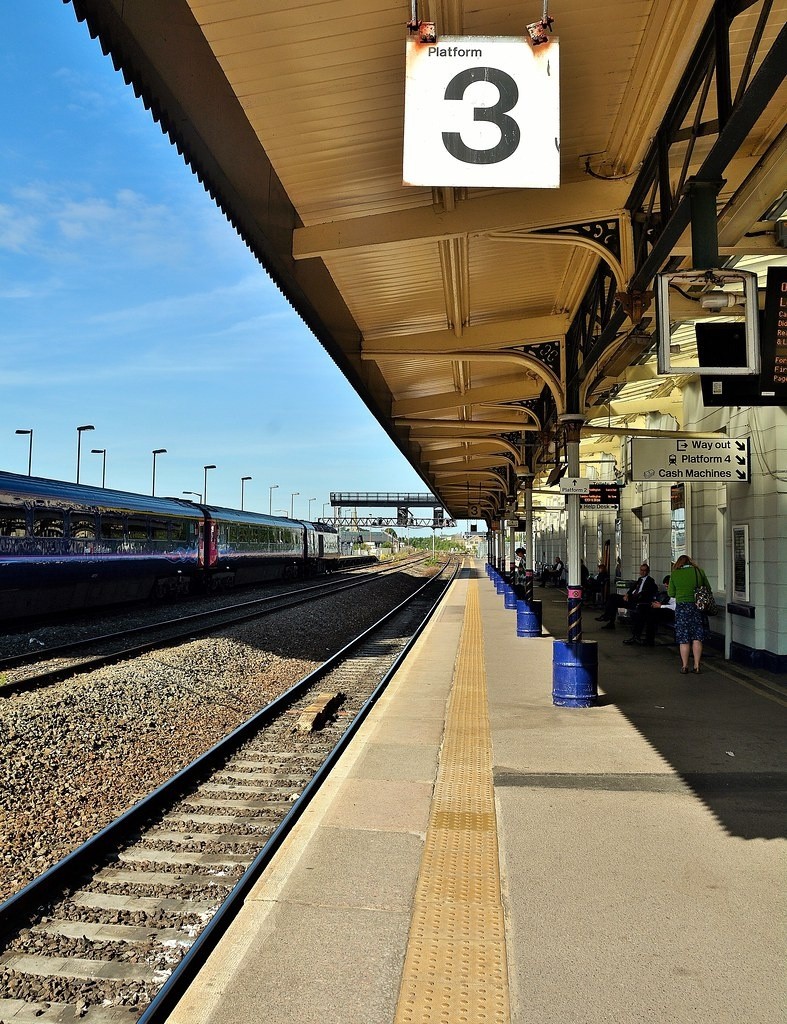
[693,566,719,617]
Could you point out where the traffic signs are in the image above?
[630,437,751,483]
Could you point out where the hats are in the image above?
[516,548,526,554]
[597,562,605,566]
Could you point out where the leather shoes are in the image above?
[601,622,617,631]
[595,615,609,621]
[641,641,656,647]
[622,636,641,645]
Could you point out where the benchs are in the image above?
[656,618,725,664]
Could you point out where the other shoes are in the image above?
[539,584,545,587]
[680,667,688,674]
[537,578,544,582]
[692,667,701,673]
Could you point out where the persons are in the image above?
[580,559,608,602]
[537,557,563,588]
[667,555,711,674]
[516,548,526,599]
[623,575,677,647]
[595,564,655,631]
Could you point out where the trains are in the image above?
[0,472,342,604]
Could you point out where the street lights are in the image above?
[276,509,288,518]
[309,498,316,522]
[344,508,350,519]
[16,429,33,476]
[152,449,167,497]
[184,491,202,505]
[269,485,279,515]
[91,449,105,488]
[203,464,215,505]
[241,477,251,511]
[292,493,299,519]
[77,425,93,485]
[322,503,329,524]
